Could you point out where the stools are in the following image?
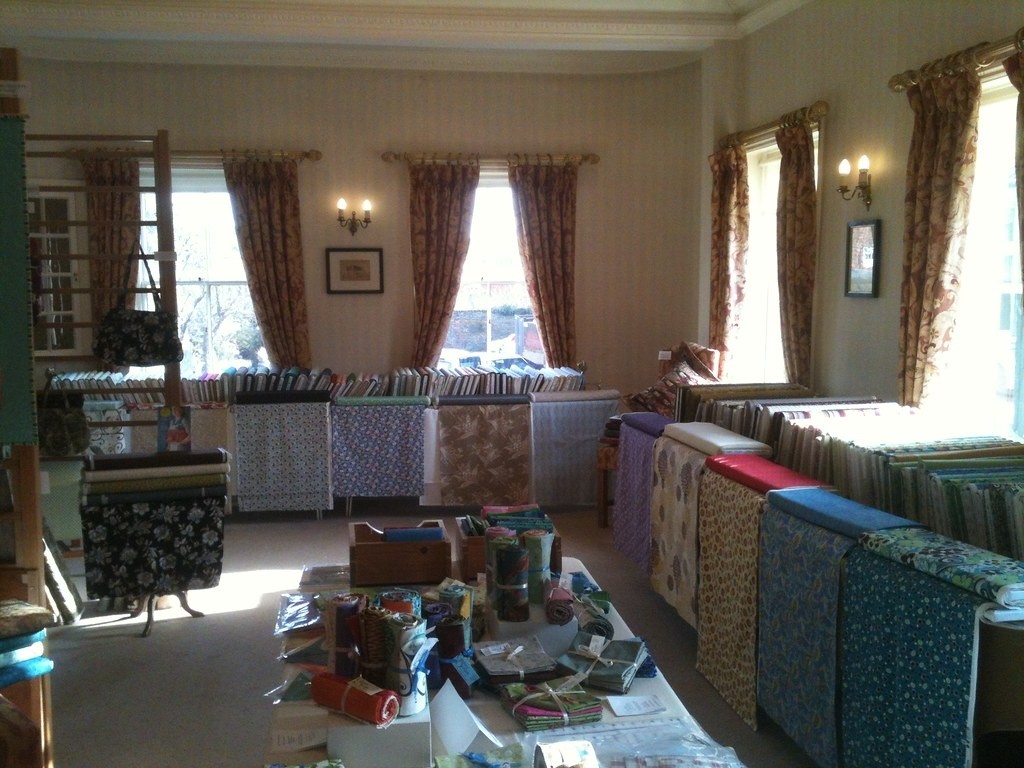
[598,445,617,527]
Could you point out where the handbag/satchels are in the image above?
[36,372,91,454]
[91,237,183,367]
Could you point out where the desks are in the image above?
[78,495,231,639]
[311,557,712,768]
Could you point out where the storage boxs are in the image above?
[349,522,451,587]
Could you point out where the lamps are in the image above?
[335,197,370,236]
[837,155,872,210]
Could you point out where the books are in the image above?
[461,503,554,536]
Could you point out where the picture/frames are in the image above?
[325,248,384,293]
[845,219,882,300]
